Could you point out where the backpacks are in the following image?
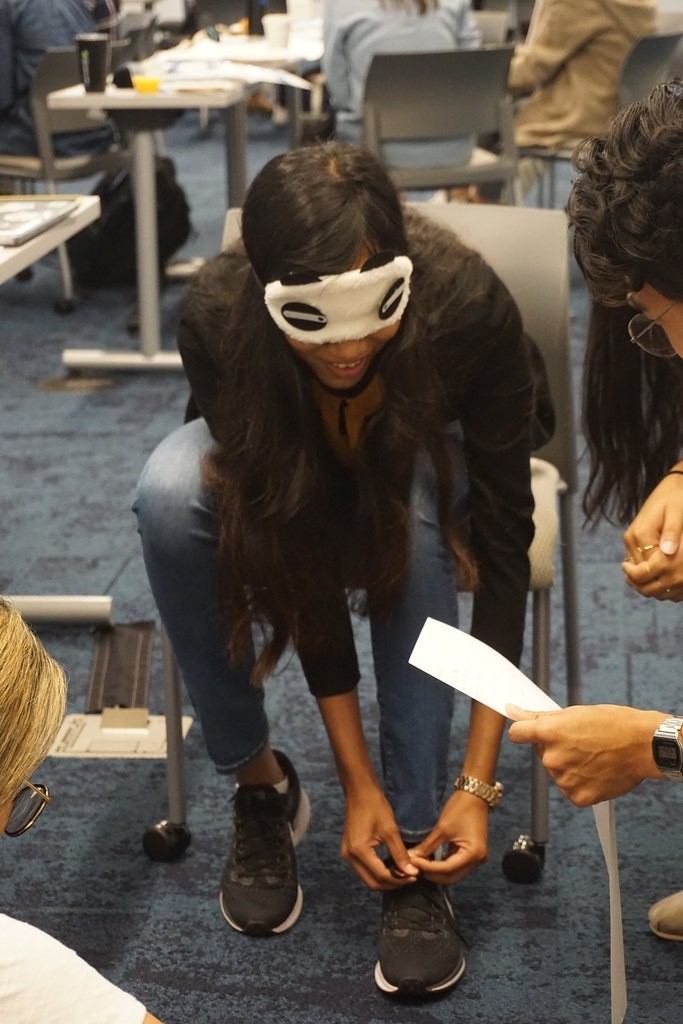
[70,157,190,291]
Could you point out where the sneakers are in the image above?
[220,750,311,939]
[374,843,465,1002]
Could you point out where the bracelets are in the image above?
[657,471,683,481]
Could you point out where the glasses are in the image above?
[4,769,52,838]
[628,303,677,358]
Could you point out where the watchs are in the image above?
[455,776,502,811]
[652,715,683,781]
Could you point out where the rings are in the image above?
[644,562,654,579]
[656,580,670,593]
[625,554,634,560]
[637,545,658,552]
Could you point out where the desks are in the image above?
[45,37,320,379]
[0,195,104,296]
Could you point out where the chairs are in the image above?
[339,48,521,205]
[143,202,581,884]
[528,33,682,209]
[0,37,135,312]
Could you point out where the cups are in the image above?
[75,33,110,93]
[261,13,289,50]
[127,62,161,93]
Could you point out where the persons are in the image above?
[130,142,557,998]
[271,0,323,127]
[508,77,683,941]
[0,599,161,1024]
[431,0,657,204]
[322,0,482,168]
[0,0,114,154]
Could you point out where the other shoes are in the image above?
[271,105,289,125]
[648,890,683,941]
